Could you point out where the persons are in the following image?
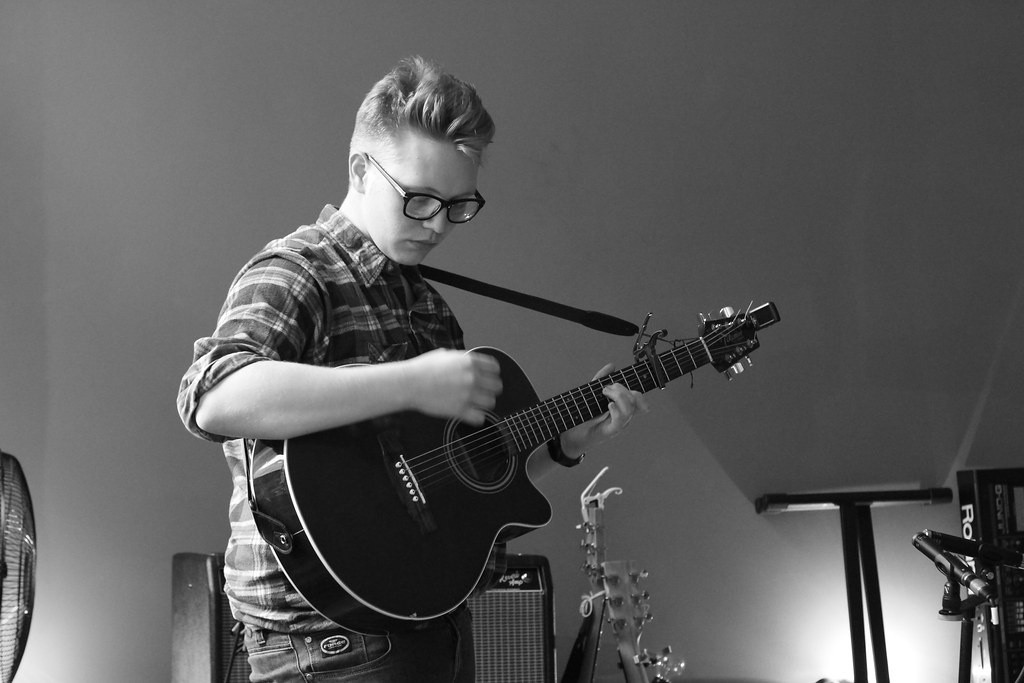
[176,58,648,683]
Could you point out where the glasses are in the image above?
[363,152,486,224]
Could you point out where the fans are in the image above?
[0,451,38,683]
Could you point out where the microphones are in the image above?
[912,528,1024,601]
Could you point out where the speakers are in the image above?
[170,551,557,683]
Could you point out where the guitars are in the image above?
[248,299,785,640]
[598,558,673,683]
[559,466,623,683]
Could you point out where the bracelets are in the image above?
[546,434,586,468]
